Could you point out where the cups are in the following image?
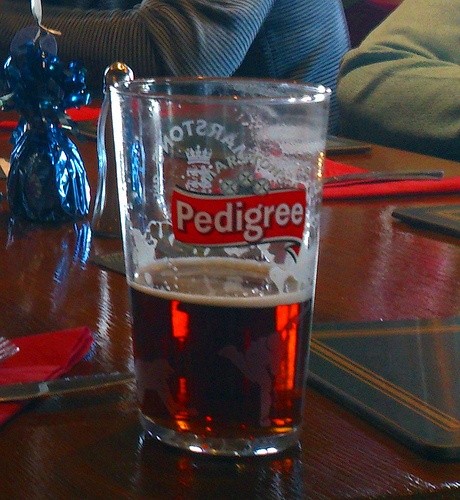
[107,74,334,462]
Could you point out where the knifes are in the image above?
[0,372,133,406]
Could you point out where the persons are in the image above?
[336,0,460,149]
[0,0,352,139]
